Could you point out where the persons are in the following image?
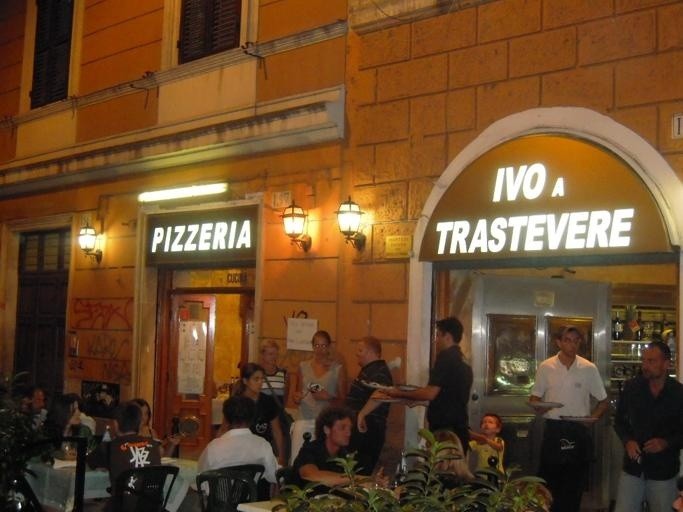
[197,396,284,503]
[468,413,505,490]
[613,342,683,512]
[379,317,473,500]
[216,363,285,465]
[260,340,288,422]
[291,331,345,418]
[341,337,393,476]
[294,409,390,488]
[529,326,609,512]
[19,387,185,512]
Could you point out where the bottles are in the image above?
[103,424,112,442]
[667,331,677,364]
[613,309,670,341]
[229,375,239,395]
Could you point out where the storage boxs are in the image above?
[611,307,676,322]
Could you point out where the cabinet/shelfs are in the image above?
[610,339,677,392]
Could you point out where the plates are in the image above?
[178,415,200,436]
[525,399,564,410]
[354,379,419,404]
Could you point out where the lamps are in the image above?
[77,221,102,263]
[334,195,367,251]
[137,182,230,203]
[278,200,312,252]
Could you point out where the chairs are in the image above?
[199,462,265,504]
[103,466,182,512]
[196,469,258,512]
